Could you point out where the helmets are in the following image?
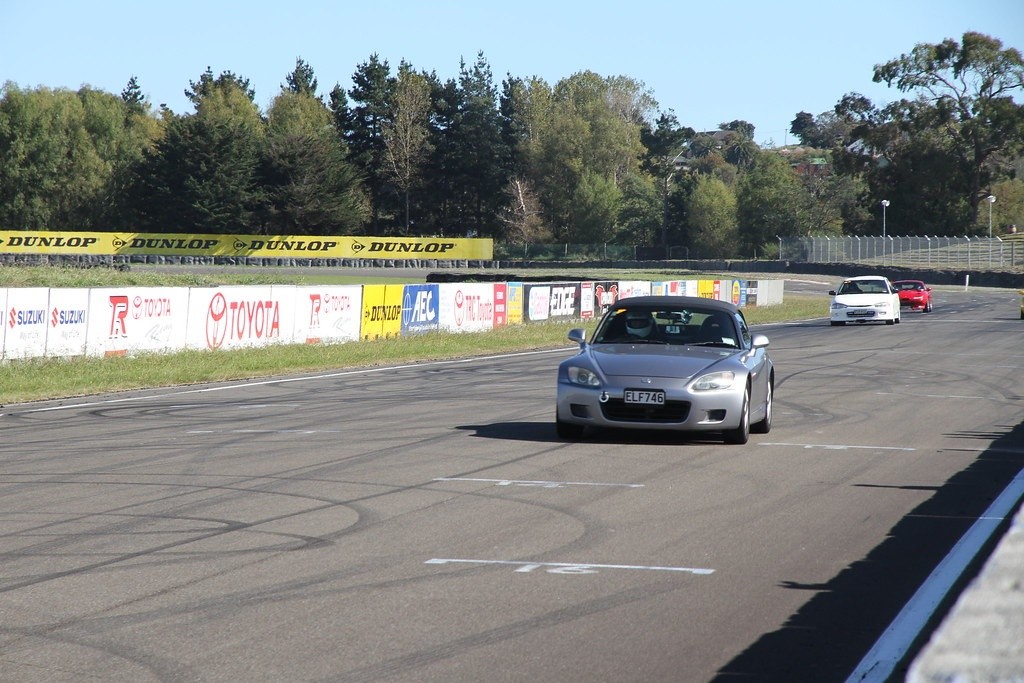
[914,284,921,288]
[848,283,858,290]
[624,308,653,338]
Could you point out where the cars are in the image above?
[891,280,932,312]
[829,275,901,325]
[555,296,776,444]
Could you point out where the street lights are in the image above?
[881,199,890,264]
[987,195,997,267]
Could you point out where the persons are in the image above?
[845,283,860,292]
[625,310,654,340]
